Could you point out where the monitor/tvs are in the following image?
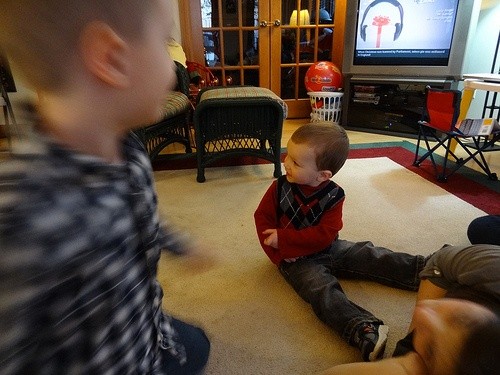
[342,0,474,82]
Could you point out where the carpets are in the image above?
[158,140,500,375]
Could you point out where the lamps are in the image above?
[289,9,310,26]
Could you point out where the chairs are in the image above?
[412,85,500,185]
[134,91,192,169]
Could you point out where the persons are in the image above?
[254,122,451,361]
[0,0,212,375]
[312,213,500,375]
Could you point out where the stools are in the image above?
[197,86,284,183]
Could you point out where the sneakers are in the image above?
[352,322,389,362]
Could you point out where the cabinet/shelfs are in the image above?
[340,79,458,141]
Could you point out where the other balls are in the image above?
[305,61,344,93]
